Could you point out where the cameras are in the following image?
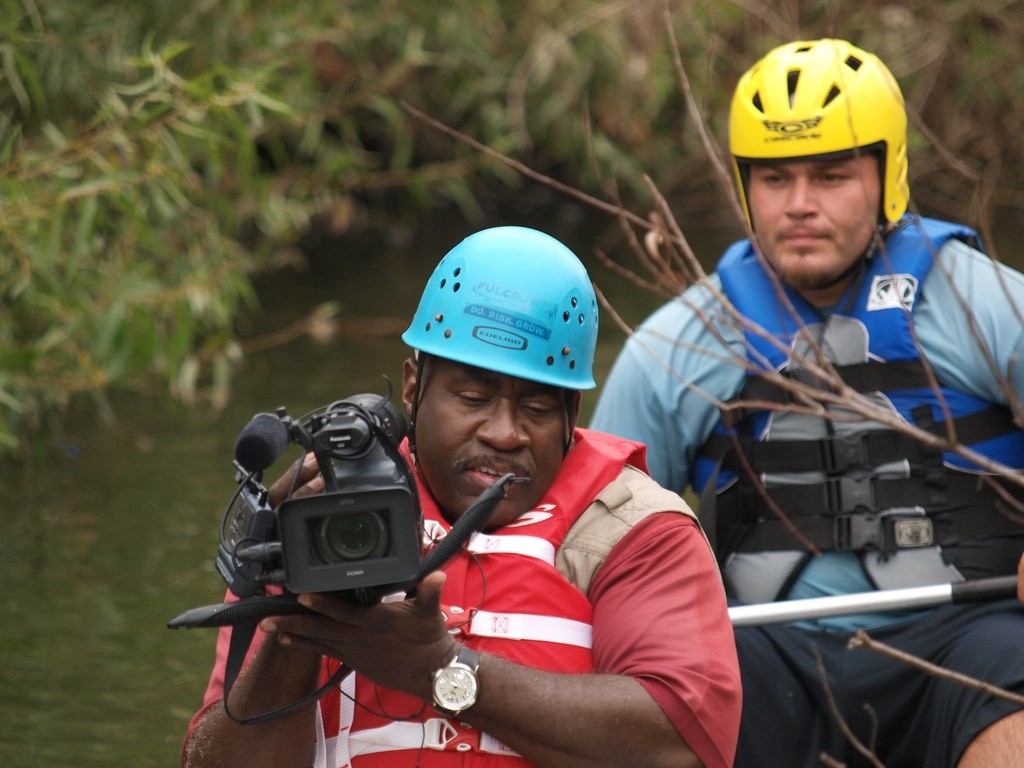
[170,375,531,626]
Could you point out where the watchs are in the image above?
[428,646,482,721]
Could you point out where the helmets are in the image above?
[731,39,908,233]
[402,226,600,394]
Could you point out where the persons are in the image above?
[584,38,1023,767]
[181,224,744,767]
